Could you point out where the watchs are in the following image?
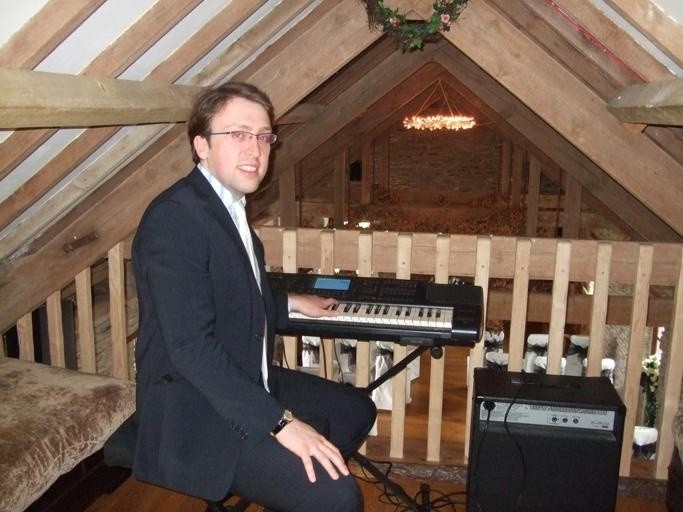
[271,408,294,435]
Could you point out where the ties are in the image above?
[232,200,272,392]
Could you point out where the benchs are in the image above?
[0,357,135,512]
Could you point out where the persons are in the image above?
[130,80,379,512]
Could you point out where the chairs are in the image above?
[302,337,410,412]
[485,330,658,460]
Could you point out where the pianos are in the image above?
[267,273,484,347]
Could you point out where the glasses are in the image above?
[211,131,278,145]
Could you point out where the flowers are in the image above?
[363,0,466,53]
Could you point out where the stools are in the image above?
[103,412,328,512]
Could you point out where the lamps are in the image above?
[403,81,477,132]
